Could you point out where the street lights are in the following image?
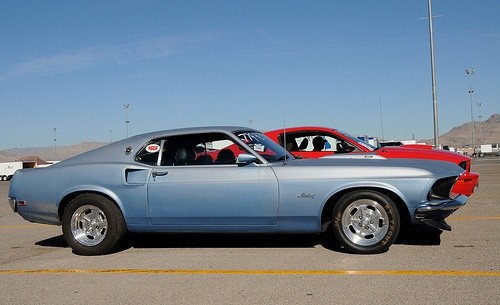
[464,68,475,146]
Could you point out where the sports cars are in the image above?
[8,125,472,257]
[191,126,480,198]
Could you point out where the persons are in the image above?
[214,148,236,164]
[313,137,325,151]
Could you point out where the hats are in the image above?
[312,136,327,145]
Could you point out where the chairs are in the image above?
[287,139,298,151]
[172,147,196,165]
[298,138,309,150]
[197,153,214,164]
